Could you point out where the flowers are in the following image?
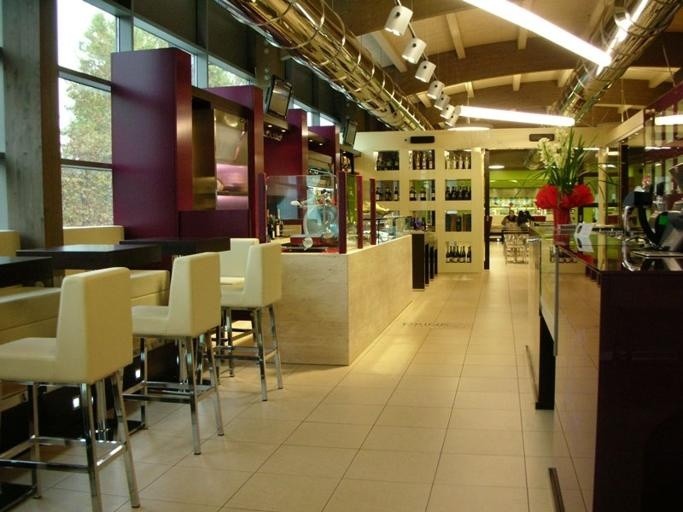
[513,128,618,207]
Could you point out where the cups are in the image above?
[657,217,683,252]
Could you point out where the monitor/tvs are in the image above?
[265,74,292,121]
[342,119,359,148]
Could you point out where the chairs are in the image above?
[502,220,532,264]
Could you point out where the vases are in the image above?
[551,205,571,230]
[552,228,569,252]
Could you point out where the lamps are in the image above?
[384,0,460,128]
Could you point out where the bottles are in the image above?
[446,244,470,263]
[455,216,461,231]
[268,218,283,239]
[569,245,623,262]
[376,156,398,170]
[408,186,434,200]
[339,151,355,225]
[375,185,398,202]
[409,216,425,232]
[445,154,469,170]
[415,153,432,170]
[445,186,471,200]
[568,226,623,245]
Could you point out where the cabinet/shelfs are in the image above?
[373,147,484,272]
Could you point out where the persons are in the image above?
[516,210,527,225]
[500,210,517,243]
[523,210,530,220]
